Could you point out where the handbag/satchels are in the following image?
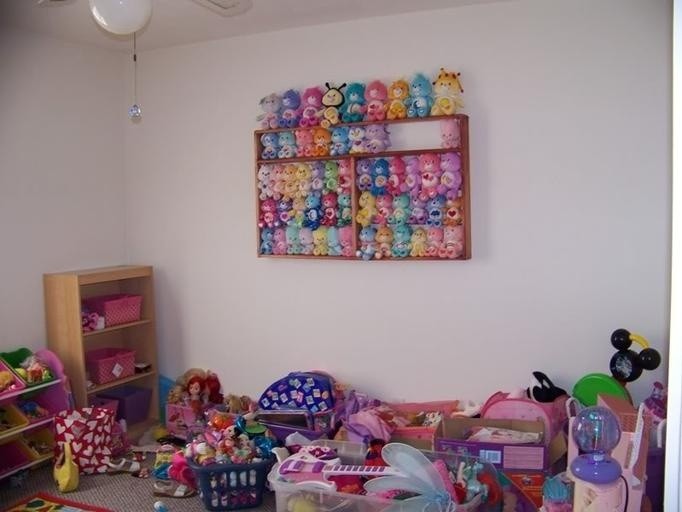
[53,407,116,492]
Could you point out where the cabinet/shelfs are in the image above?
[254,113,470,262]
[43,263,160,440]
[1,349,74,478]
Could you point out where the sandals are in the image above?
[106,458,143,475]
[152,480,195,498]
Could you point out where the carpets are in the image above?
[2,493,113,512]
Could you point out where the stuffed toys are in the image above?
[296,87,325,128]
[260,132,279,160]
[256,93,281,130]
[428,68,464,116]
[278,89,301,128]
[278,131,296,159]
[383,80,409,120]
[329,127,350,156]
[313,82,347,128]
[355,151,464,260]
[347,125,368,154]
[364,124,391,154]
[167,367,223,419]
[440,118,461,149]
[404,74,434,119]
[188,394,276,505]
[295,129,313,157]
[311,129,332,157]
[338,82,366,123]
[258,160,354,257]
[360,80,388,121]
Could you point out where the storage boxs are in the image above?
[90,383,153,426]
[160,389,664,512]
[85,347,136,383]
[88,292,142,327]
